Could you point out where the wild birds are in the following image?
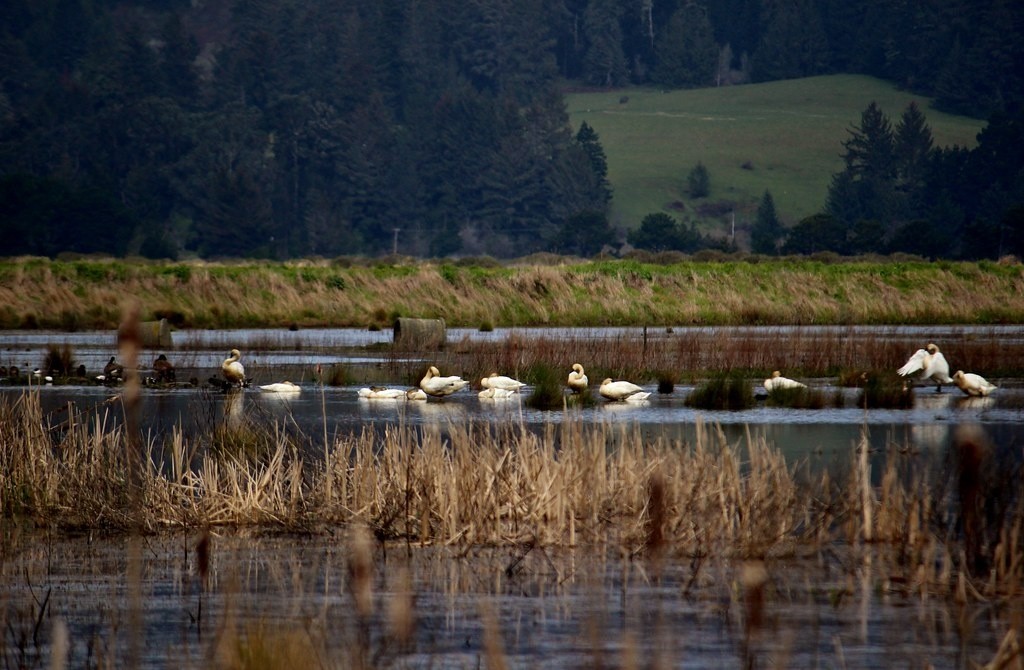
[217,349,246,389]
[896,343,999,396]
[598,378,653,400]
[258,380,301,392]
[357,365,527,400]
[568,363,588,395]
[763,370,808,396]
